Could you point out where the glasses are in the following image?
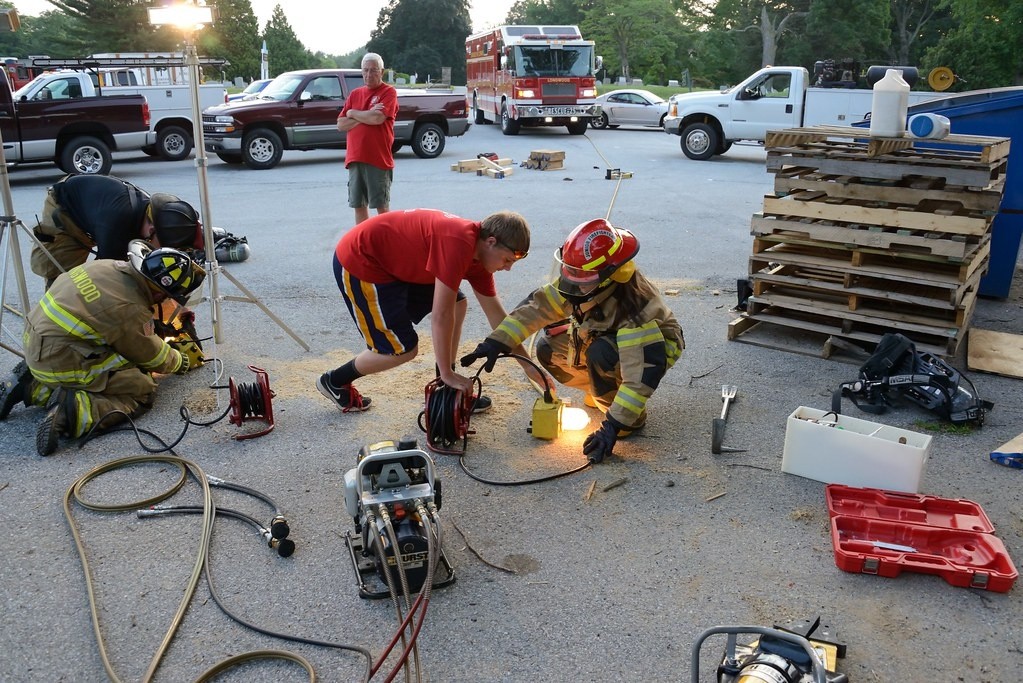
[492,235,528,259]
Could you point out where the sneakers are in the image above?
[316,368,372,411]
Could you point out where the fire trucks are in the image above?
[465,24,603,136]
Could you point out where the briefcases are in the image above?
[827,482,1020,595]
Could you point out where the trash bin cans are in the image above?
[852,85,1023,301]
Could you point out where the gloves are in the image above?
[460,341,500,373]
[582,420,618,462]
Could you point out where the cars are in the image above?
[590,88,669,129]
[227,78,275,100]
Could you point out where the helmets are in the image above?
[150,192,199,249]
[559,218,640,285]
[128,239,207,304]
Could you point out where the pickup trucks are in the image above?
[664,63,958,162]
[201,68,473,171]
[0,65,157,176]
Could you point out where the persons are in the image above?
[336,53,400,224]
[767,72,789,98]
[594,57,607,82]
[0,239,206,457]
[29,173,200,331]
[310,84,323,96]
[316,207,556,410]
[461,220,687,465]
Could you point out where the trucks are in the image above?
[12,59,226,161]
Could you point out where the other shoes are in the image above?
[0,359,31,420]
[35,385,71,457]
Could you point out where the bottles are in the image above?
[869,69,910,138]
[908,112,951,140]
